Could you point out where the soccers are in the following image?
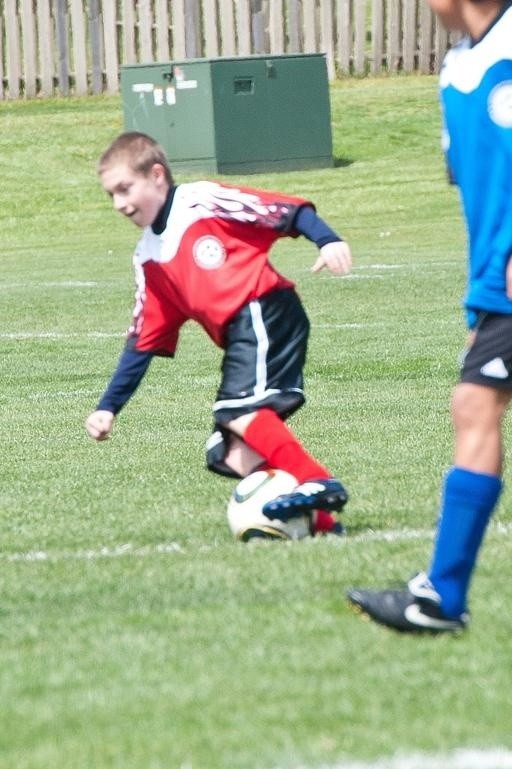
[227,468,311,542]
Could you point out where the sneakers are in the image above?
[262,479,347,517]
[346,574,471,641]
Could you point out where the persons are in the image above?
[342,0,512,638]
[83,131,355,538]
